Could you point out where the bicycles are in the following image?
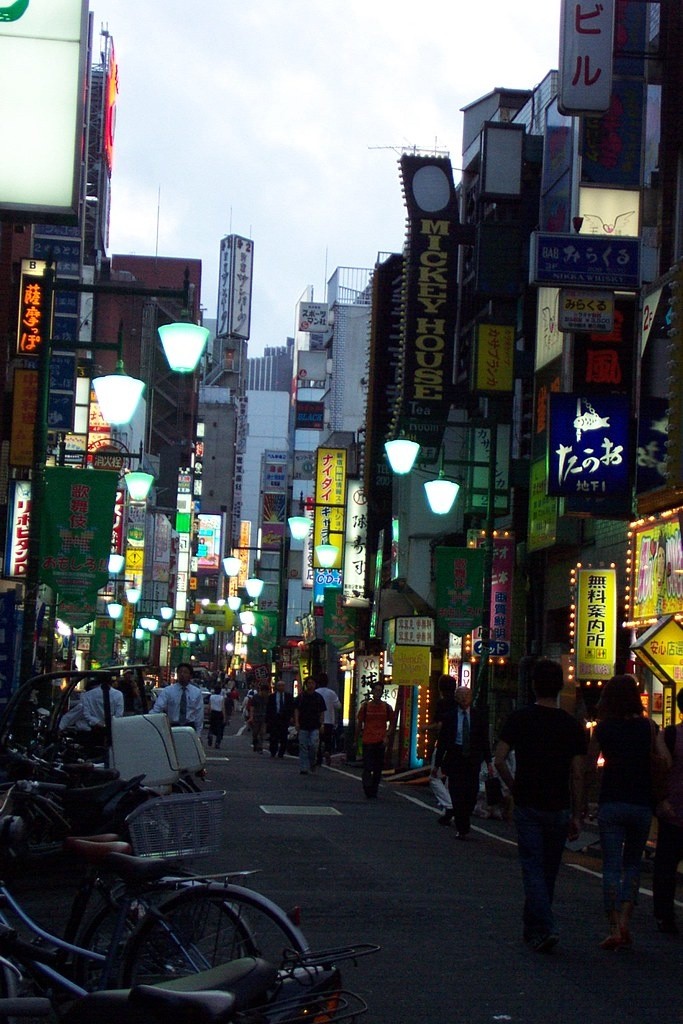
[0,664,383,1023]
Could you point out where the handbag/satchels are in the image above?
[646,718,673,800]
[428,776,453,809]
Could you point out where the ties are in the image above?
[179,686,187,726]
[462,710,470,756]
[279,692,284,712]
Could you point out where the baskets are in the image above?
[124,789,228,860]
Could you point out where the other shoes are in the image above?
[619,925,633,945]
[366,793,377,801]
[207,734,213,747]
[438,816,451,826]
[656,915,676,931]
[301,769,309,775]
[536,932,560,953]
[523,927,540,947]
[455,830,473,841]
[600,936,623,950]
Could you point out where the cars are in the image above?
[201,691,213,718]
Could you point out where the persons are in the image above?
[352,683,396,801]
[492,660,683,952]
[53,656,342,774]
[430,687,497,839]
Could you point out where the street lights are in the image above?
[287,491,362,762]
[16,261,211,695]
[383,418,498,738]
[41,427,155,711]
[219,534,292,642]
